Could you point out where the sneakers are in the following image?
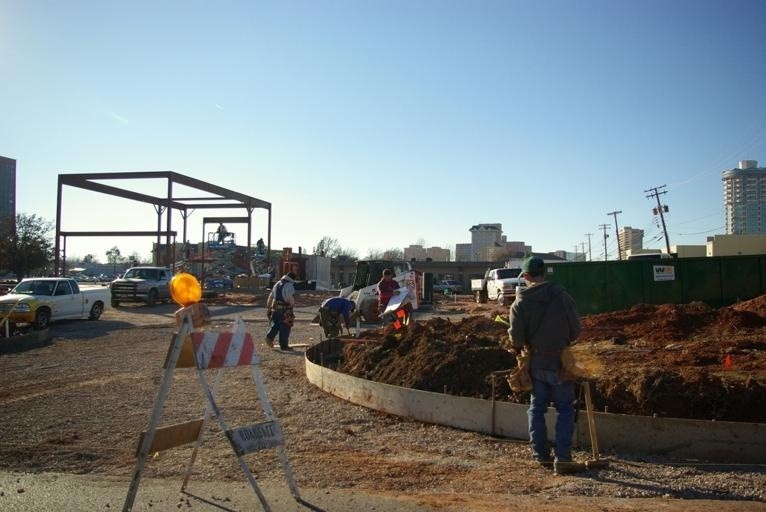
[264,336,274,350]
[535,456,556,465]
[553,458,587,476]
[279,344,294,351]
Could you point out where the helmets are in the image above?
[167,271,203,309]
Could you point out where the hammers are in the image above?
[583,383,609,470]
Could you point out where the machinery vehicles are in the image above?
[310,258,433,327]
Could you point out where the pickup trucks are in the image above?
[110,267,202,307]
[471,268,527,306]
[0,277,113,328]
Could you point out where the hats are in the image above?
[350,300,356,313]
[517,254,546,281]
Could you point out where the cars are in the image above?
[433,280,465,293]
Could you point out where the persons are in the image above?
[376,268,402,329]
[215,223,227,245]
[320,297,355,339]
[507,256,586,473]
[265,271,297,351]
[257,238,264,246]
